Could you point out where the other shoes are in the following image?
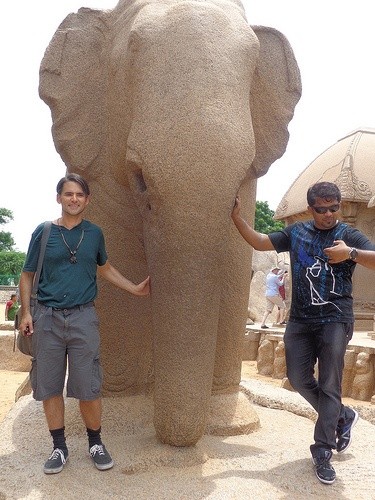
[280,321,286,324]
[261,324,269,328]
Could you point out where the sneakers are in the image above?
[44,447,68,473]
[336,406,359,453]
[88,443,113,470]
[312,451,336,484]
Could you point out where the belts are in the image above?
[53,301,95,310]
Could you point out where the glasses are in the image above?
[311,203,339,214]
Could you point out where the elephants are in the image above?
[38,0,303,449]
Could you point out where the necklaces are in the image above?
[57,218,84,264]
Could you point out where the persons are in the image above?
[5,294,20,321]
[231,182,375,485]
[20,173,151,474]
[261,267,288,329]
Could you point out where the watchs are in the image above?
[349,247,359,261]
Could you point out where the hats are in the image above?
[271,266,281,271]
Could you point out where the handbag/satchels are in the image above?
[14,305,35,357]
